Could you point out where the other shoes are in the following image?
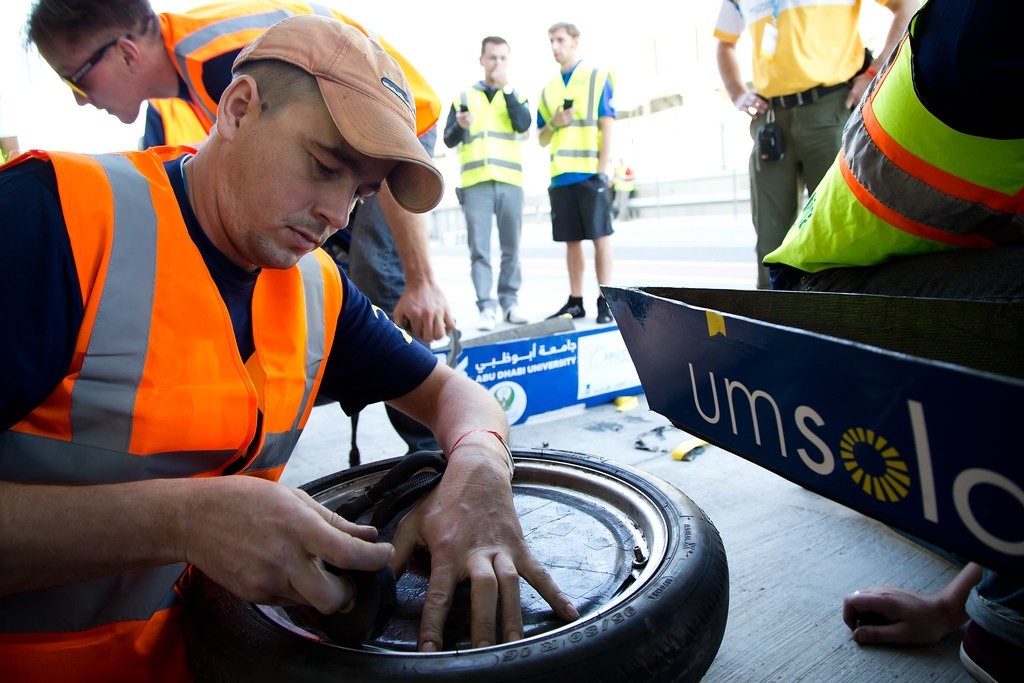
[959,620,1024,683]
[546,304,586,321]
[597,296,612,323]
[477,308,497,330]
[502,306,528,323]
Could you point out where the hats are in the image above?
[229,14,444,213]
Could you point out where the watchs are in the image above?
[503,84,514,95]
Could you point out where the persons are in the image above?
[28,0,456,456]
[537,21,618,324]
[0,15,580,683]
[444,37,532,331]
[713,0,1024,683]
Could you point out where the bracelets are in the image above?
[548,120,559,132]
[865,67,878,79]
[753,102,759,108]
[449,429,516,481]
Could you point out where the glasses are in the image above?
[60,34,132,98]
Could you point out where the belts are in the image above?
[770,82,847,107]
